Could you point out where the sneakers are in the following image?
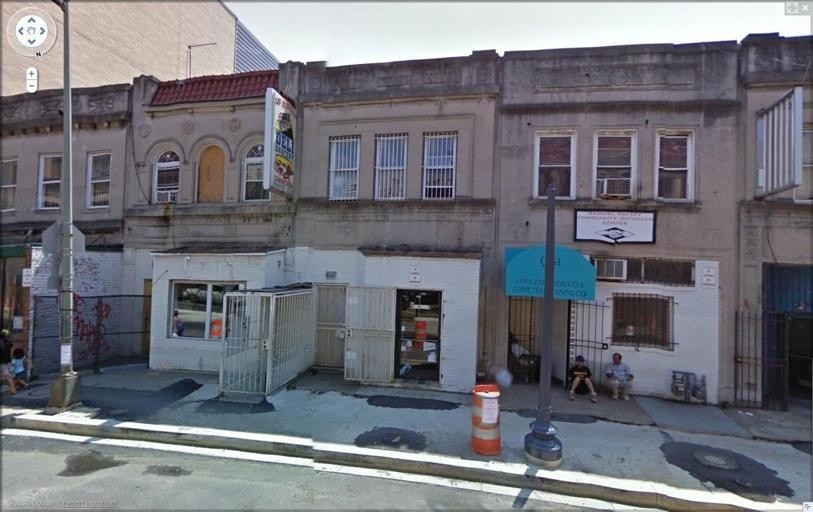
[590,395,597,402]
[622,389,629,401]
[568,392,576,400]
[612,390,618,400]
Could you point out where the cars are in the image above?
[613,320,649,338]
[183,284,239,307]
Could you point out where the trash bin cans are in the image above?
[471,383,502,456]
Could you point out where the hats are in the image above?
[576,356,584,361]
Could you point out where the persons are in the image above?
[0,332,30,395]
[173,310,182,335]
[511,335,540,381]
[605,353,634,400]
[568,355,597,403]
